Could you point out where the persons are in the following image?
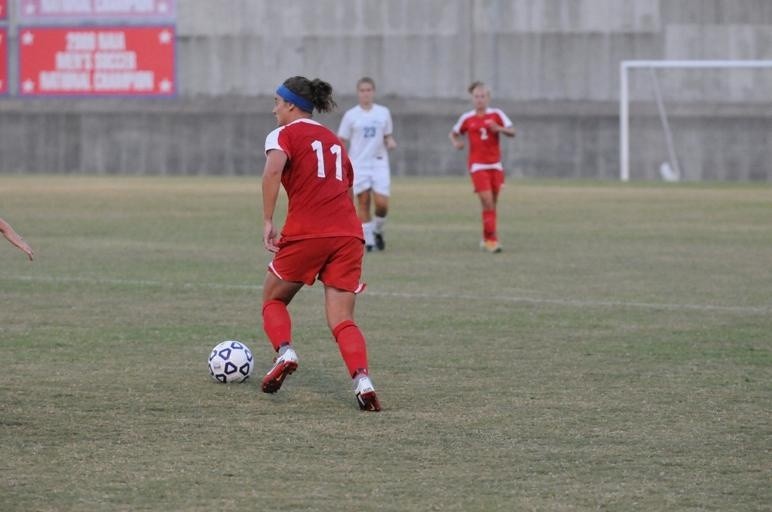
[259,75,383,412]
[335,77,399,254]
[448,80,519,252]
[0,216,35,263]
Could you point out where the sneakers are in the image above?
[354,376,382,411]
[261,349,299,394]
[366,234,385,252]
[480,240,502,253]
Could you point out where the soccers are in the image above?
[207,340,253,383]
